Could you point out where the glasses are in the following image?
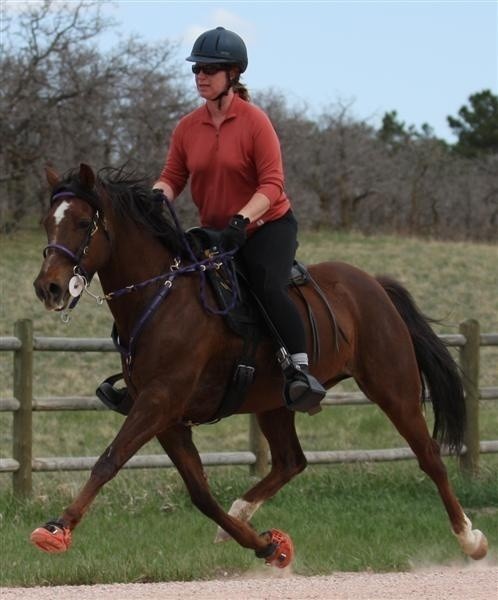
[192,64,226,74]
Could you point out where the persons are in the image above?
[96,28,325,416]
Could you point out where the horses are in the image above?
[29,162,491,570]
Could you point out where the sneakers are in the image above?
[288,380,323,416]
[100,383,133,415]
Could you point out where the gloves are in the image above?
[220,214,250,244]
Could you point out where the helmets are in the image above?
[185,27,248,73]
